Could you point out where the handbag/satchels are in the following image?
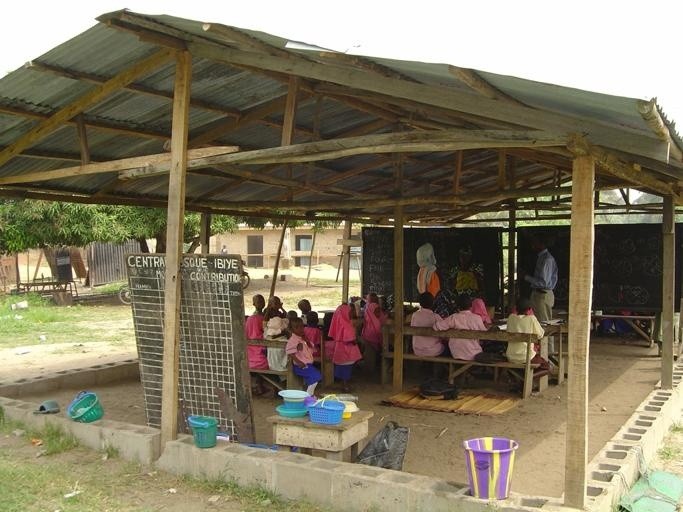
[475,351,506,364]
[356,421,409,470]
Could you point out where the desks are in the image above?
[18,281,78,304]
[265,411,375,462]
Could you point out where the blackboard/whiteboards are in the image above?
[124,252,256,445]
[361,226,501,309]
[517,222,683,311]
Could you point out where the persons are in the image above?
[517,234,559,366]
[409,273,499,384]
[415,242,441,308]
[446,248,479,294]
[244,291,394,398]
[505,296,554,395]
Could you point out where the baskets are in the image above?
[68,393,104,423]
[308,394,345,425]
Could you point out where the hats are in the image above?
[267,316,290,336]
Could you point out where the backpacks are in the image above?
[420,378,461,399]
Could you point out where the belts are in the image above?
[536,288,546,293]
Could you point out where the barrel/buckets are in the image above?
[188,416,218,448]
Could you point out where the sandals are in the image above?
[344,384,353,393]
[256,386,267,394]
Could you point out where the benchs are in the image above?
[245,310,338,393]
[590,315,656,349]
[381,324,569,399]
